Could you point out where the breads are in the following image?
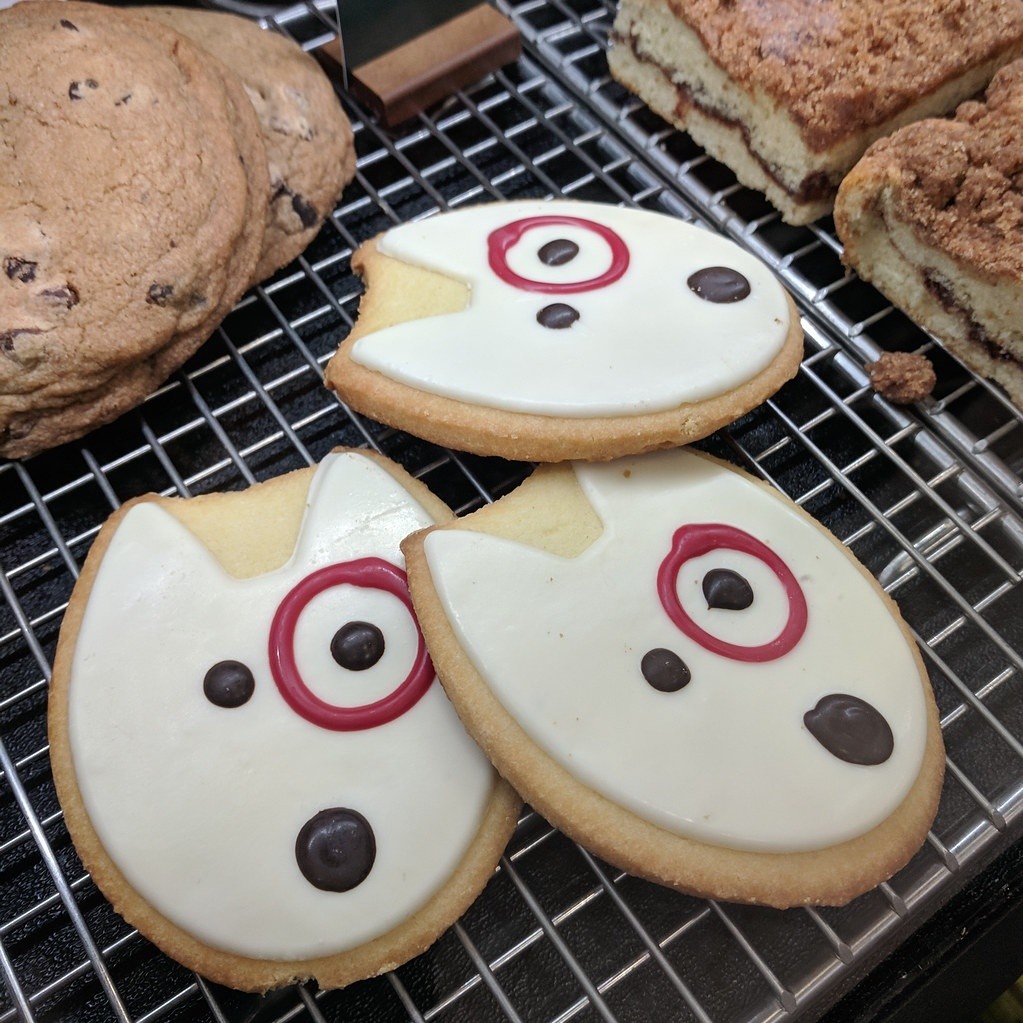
[605,0,1023,411]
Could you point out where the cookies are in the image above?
[1,1,948,993]
